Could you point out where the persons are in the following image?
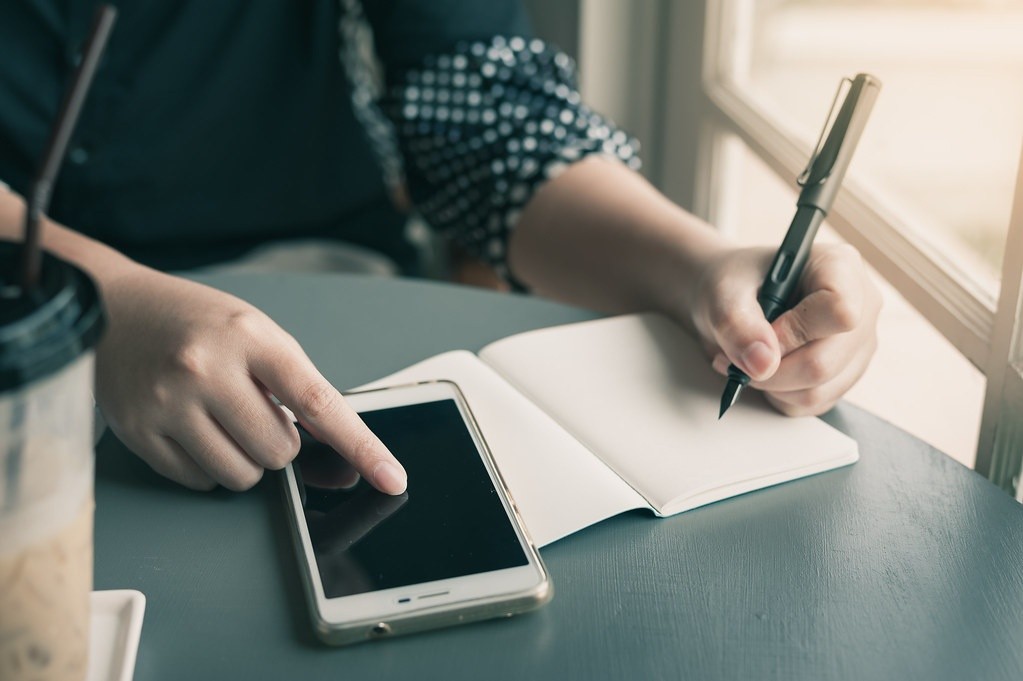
[0,0,881,496]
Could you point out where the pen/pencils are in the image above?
[719,70,885,419]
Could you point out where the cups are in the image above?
[0,234,110,681]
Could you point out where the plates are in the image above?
[90,588,146,681]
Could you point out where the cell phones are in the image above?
[264,380,555,648]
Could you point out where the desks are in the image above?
[93,268,1023,681]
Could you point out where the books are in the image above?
[343,310,863,549]
